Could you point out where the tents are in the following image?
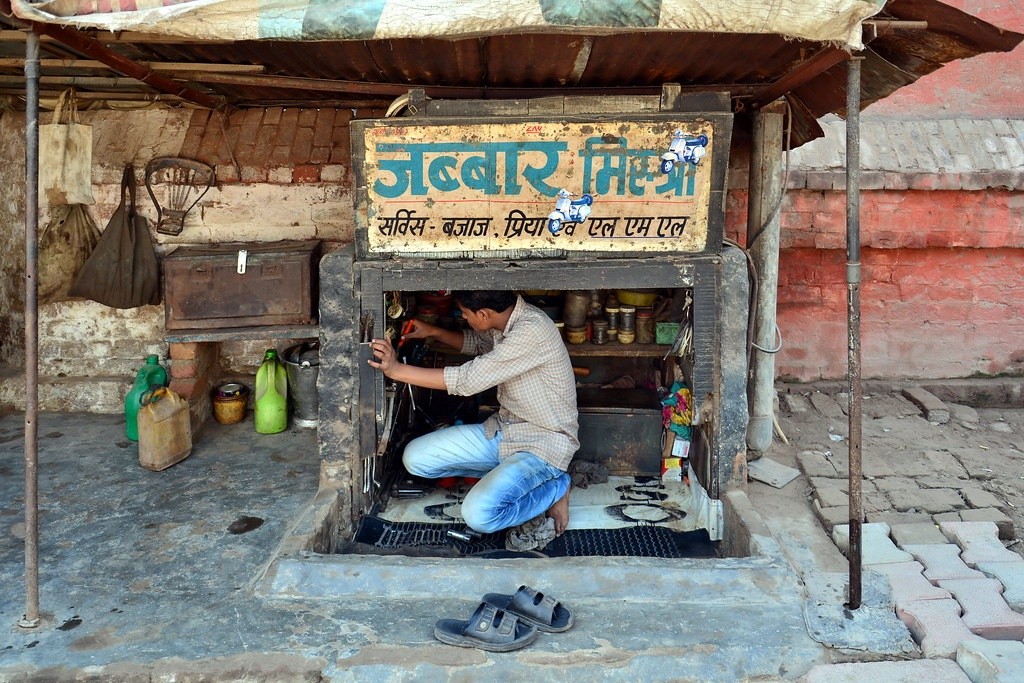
[6,0,1024,630]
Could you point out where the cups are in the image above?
[554,294,653,345]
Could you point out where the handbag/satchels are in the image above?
[36,87,97,206]
[68,163,161,310]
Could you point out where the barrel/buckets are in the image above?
[281,341,319,427]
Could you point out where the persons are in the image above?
[367,290,581,539]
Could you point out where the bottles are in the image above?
[254,349,287,433]
[124,355,167,442]
[137,388,192,471]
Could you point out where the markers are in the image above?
[397,319,413,353]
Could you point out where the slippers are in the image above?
[434,601,538,652]
[481,585,574,633]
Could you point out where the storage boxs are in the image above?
[476,386,661,479]
[155,238,324,329]
[656,322,680,344]
